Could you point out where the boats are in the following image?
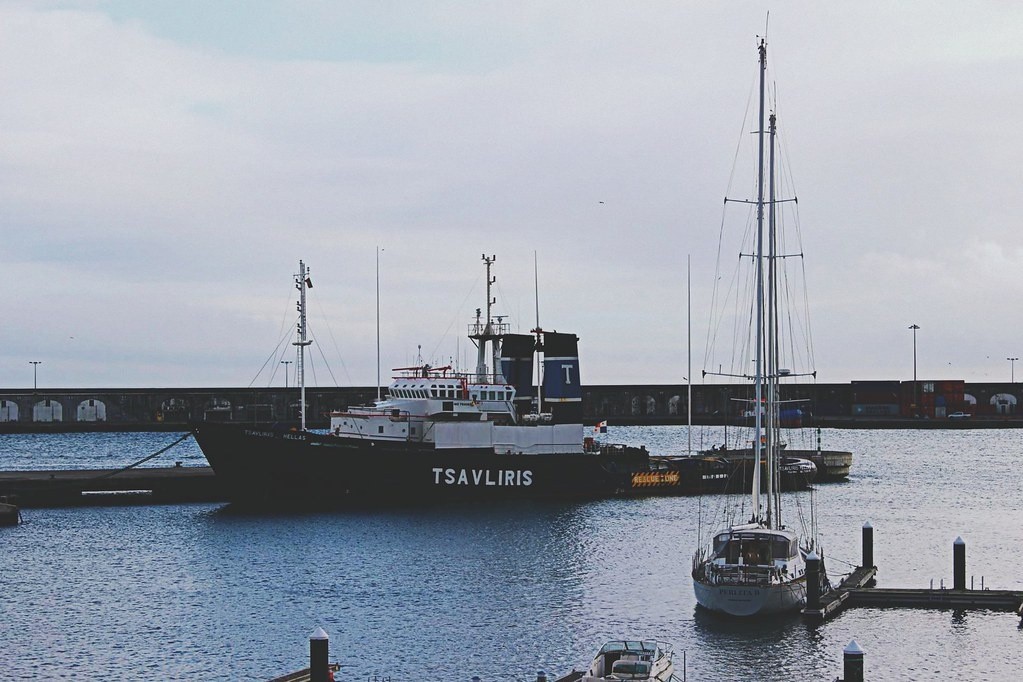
[536,638,687,682]
[696,428,858,484]
[186,242,818,518]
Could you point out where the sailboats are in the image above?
[691,9,829,625]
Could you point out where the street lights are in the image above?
[909,323,921,405]
[29,362,41,394]
[1006,357,1018,381]
[282,360,293,387]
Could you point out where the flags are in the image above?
[593,420,607,433]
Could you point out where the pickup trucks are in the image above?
[948,411,972,420]
